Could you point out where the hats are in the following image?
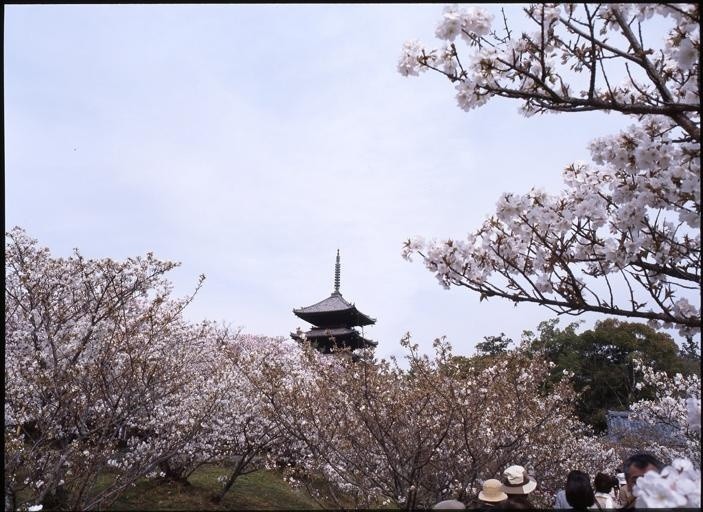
[478,479,507,502]
[499,465,537,494]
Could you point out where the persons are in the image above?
[432,453,663,510]
